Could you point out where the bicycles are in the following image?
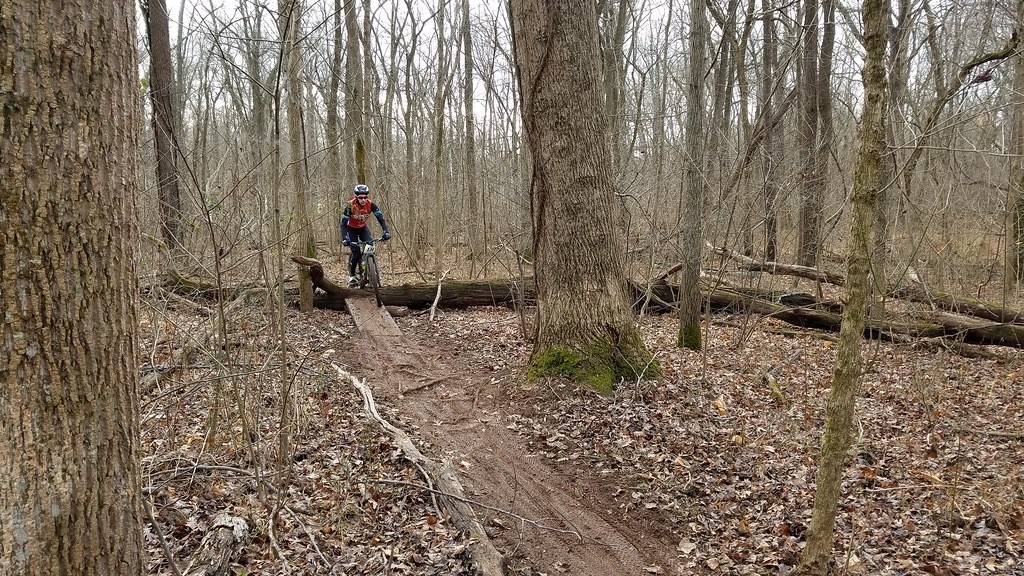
[342,231,390,308]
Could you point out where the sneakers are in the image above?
[349,276,357,288]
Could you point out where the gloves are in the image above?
[343,239,351,246]
[382,233,391,240]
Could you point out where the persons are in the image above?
[341,184,391,287]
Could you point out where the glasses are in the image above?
[357,197,368,199]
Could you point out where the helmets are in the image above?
[354,185,370,195]
